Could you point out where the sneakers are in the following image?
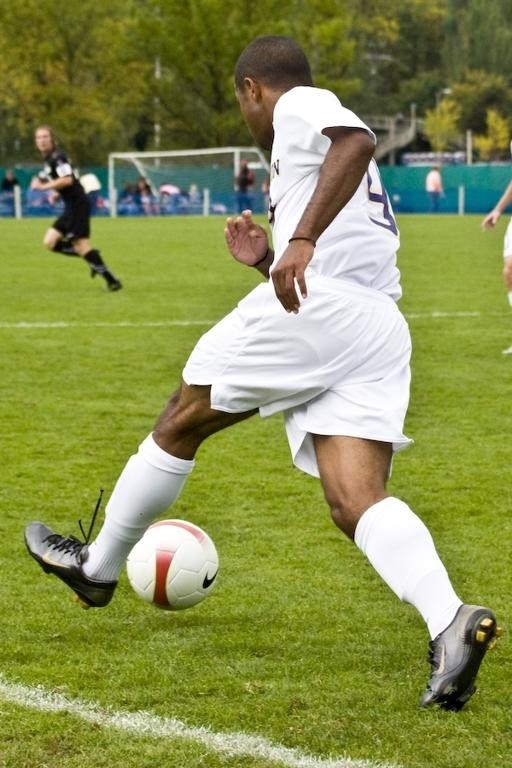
[25,522,118,609]
[423,603,503,712]
[91,250,121,290]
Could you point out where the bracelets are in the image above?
[288,237,317,247]
[254,248,270,265]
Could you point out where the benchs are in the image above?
[0,190,203,217]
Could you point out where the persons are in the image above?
[1,159,270,215]
[30,126,121,292]
[426,166,442,213]
[483,182,511,353]
[23,35,504,712]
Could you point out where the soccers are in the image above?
[126,519,219,610]
